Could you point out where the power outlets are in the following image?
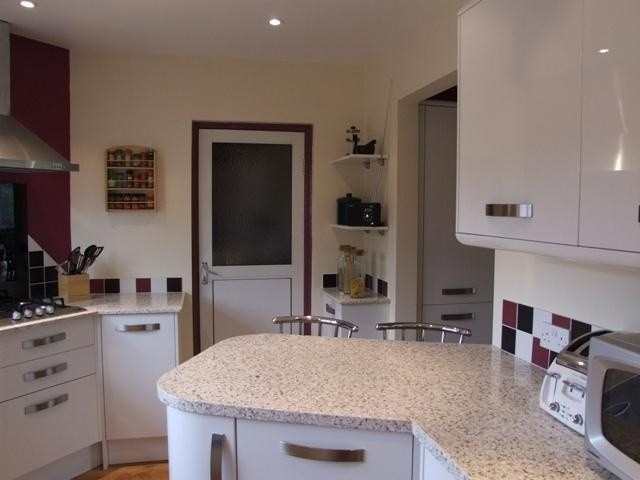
[539,321,570,354]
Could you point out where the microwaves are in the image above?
[585,332,640,478]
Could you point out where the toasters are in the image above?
[539,329,619,437]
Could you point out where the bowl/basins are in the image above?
[354,145,375,154]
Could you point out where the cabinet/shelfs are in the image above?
[330,154,389,236]
[321,290,390,340]
[235,418,414,480]
[104,144,157,212]
[454,0,640,270]
[165,406,237,480]
[0,312,105,480]
[98,312,182,471]
[418,99,493,346]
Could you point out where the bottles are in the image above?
[336,245,366,300]
[336,193,362,225]
[107,148,154,209]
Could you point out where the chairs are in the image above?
[375,322,472,344]
[272,315,359,339]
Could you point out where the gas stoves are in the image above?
[0,297,87,331]
